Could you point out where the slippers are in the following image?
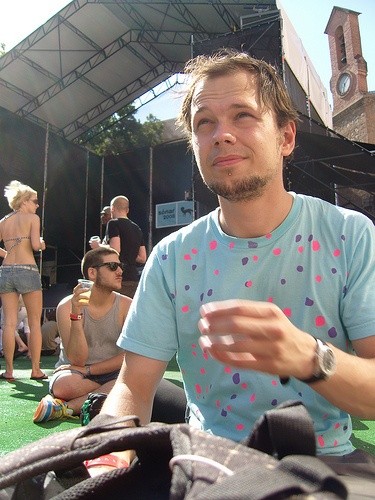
[30,373,48,379]
[15,345,28,358]
[0,372,15,381]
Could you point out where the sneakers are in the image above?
[81,391,108,426]
[33,394,80,424]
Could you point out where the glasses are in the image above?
[93,261,126,272]
[31,199,39,204]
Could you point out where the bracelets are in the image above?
[84,366,91,378]
[84,454,128,470]
[69,314,83,321]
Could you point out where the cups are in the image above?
[91,235,99,240]
[39,237,43,251]
[78,278,95,305]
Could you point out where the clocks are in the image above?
[335,71,354,98]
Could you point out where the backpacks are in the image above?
[0,399,352,500]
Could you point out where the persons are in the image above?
[31,247,135,422]
[89,195,147,282]
[83,49,375,500]
[0,180,48,380]
[0,295,72,359]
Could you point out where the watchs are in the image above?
[302,336,337,384]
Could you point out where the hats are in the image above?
[100,206,110,215]
